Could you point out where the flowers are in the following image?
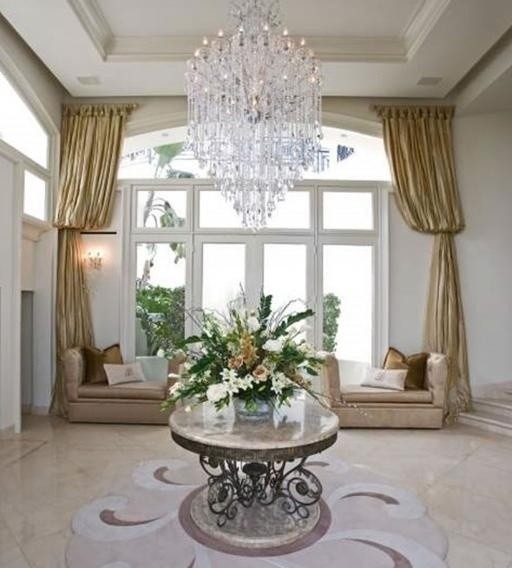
[156,283,374,426]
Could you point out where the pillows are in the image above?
[360,346,431,389]
[84,343,147,385]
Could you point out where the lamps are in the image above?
[185,1,324,235]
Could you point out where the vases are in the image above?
[233,391,277,422]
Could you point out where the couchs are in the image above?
[60,349,187,425]
[315,348,448,430]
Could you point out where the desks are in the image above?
[167,398,340,549]
